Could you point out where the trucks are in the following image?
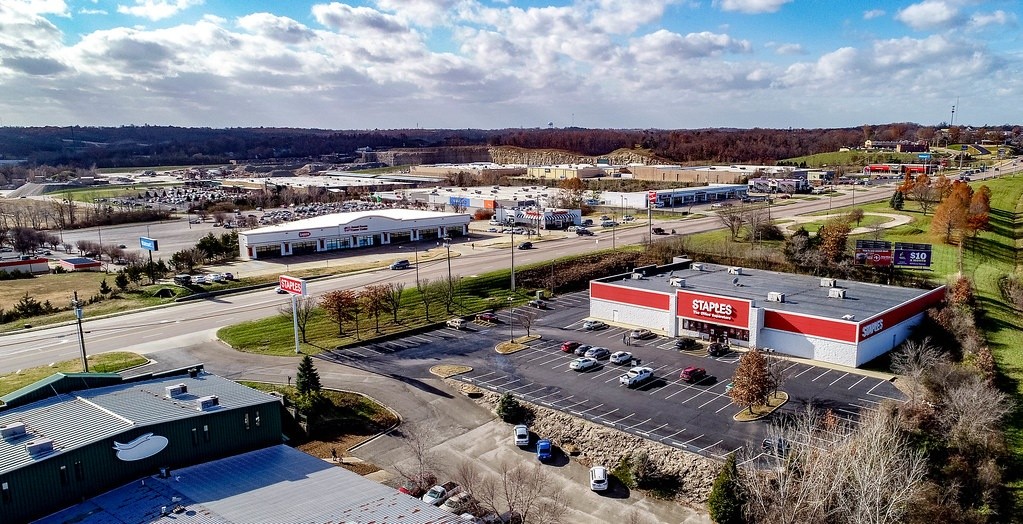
[652,227,665,234]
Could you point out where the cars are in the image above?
[172,271,235,286]
[95,189,228,209]
[526,300,547,309]
[0,244,127,265]
[517,242,533,251]
[838,152,1023,186]
[475,312,499,323]
[711,193,775,210]
[724,381,739,393]
[188,200,428,229]
[397,471,437,498]
[561,319,730,383]
[430,183,565,200]
[459,502,496,522]
[62,197,70,205]
[476,506,522,524]
[486,202,634,236]
[761,437,791,456]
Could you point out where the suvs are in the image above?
[446,319,467,331]
[388,259,409,270]
[513,424,530,446]
[536,439,553,462]
[589,465,609,491]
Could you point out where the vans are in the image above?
[439,490,474,515]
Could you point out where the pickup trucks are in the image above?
[619,366,655,388]
[421,480,460,506]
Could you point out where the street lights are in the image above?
[507,297,515,343]
